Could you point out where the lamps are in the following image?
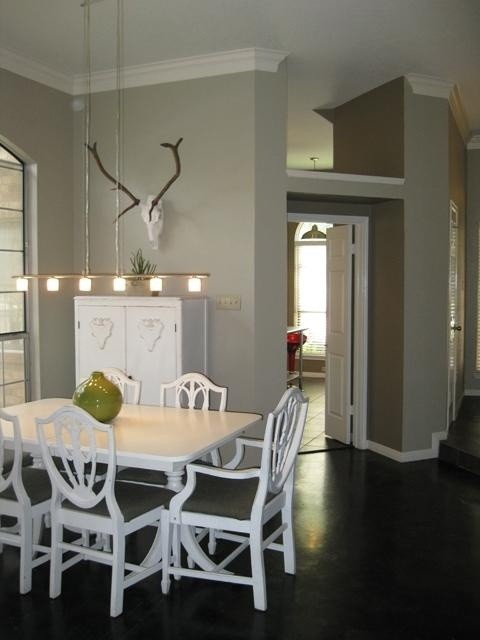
[12,0,210,292]
[300,157,326,239]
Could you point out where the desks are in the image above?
[287,326,310,392]
[0,398,262,492]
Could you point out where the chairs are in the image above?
[35,404,178,617]
[287,333,307,388]
[161,385,310,611]
[52,367,142,485]
[73,296,209,409]
[115,373,227,488]
[0,409,88,595]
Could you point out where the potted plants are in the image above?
[126,248,156,296]
